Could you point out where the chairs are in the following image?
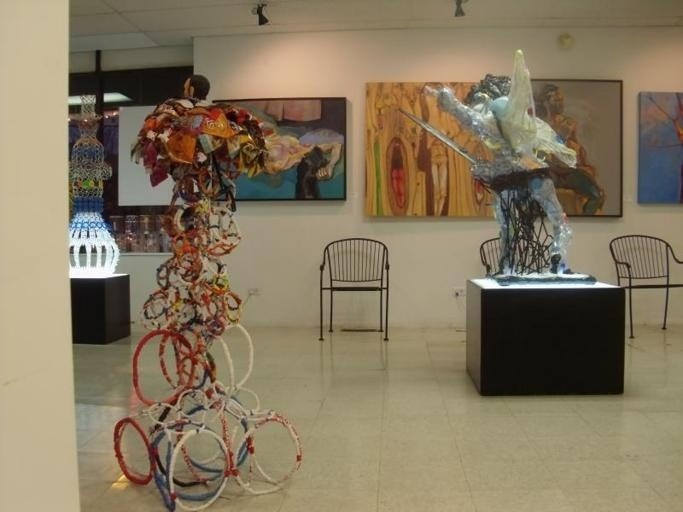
[318,238,389,342]
[479,237,542,277]
[608,235,683,339]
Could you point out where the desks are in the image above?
[72,275,129,344]
[466,275,625,395]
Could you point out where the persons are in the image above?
[540,84,605,216]
[183,73,210,100]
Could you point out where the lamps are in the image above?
[251,1,271,28]
